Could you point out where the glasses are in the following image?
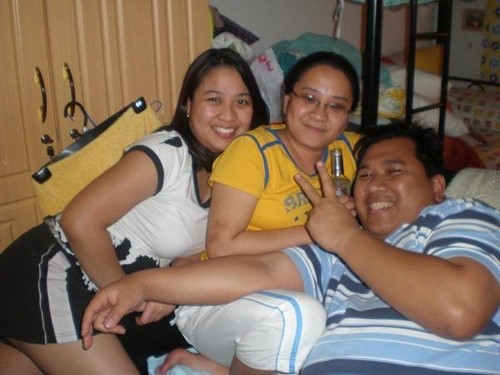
[290,89,352,116]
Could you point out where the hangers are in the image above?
[31,96,163,185]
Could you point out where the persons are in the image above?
[1,47,270,375]
[81,120,500,374]
[154,50,370,374]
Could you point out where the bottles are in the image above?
[330,148,350,197]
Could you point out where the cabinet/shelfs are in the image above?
[0,0,212,253]
